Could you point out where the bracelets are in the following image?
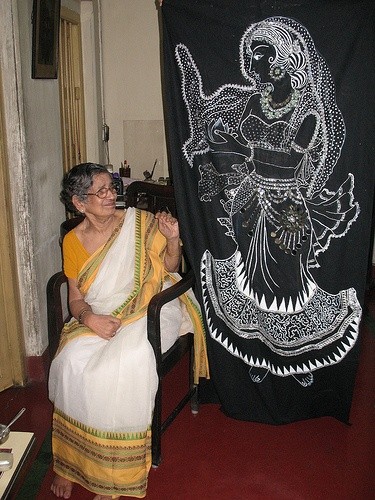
[75,307,92,324]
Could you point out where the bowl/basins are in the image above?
[0,424,10,444]
[119,168,131,177]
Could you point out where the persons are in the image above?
[48,162,210,499]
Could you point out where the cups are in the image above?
[0,453,13,472]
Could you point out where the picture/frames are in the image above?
[31,0,61,80]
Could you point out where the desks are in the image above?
[0,424,36,500]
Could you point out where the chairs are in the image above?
[47,183,210,470]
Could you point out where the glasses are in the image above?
[80,185,119,199]
